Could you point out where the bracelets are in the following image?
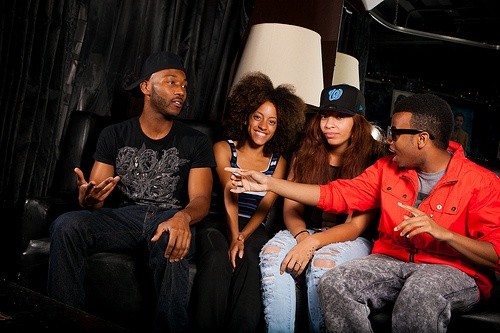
[294,230,309,239]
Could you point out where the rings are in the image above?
[297,263,301,267]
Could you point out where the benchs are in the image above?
[22,111,500,333]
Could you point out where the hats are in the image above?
[126,51,186,91]
[318,84,364,115]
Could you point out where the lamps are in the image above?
[228,22,324,108]
[332,52,360,90]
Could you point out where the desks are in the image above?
[0,276,128,333]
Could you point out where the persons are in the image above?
[188,73,306,333]
[450,113,469,145]
[45,50,216,333]
[224,92,500,333]
[259,84,386,333]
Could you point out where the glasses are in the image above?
[389,126,434,143]
